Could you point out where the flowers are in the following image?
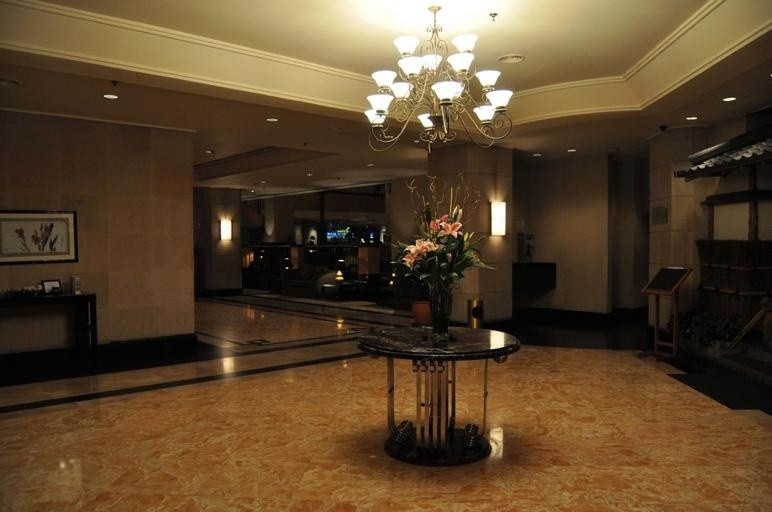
[404,205,496,292]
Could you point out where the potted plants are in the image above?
[390,209,433,326]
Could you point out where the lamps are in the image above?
[491,201,506,236]
[363,5,513,153]
[218,219,234,241]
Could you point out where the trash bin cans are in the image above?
[468,299,483,328]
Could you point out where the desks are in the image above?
[0,293,98,376]
[357,326,521,467]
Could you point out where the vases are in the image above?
[429,287,451,341]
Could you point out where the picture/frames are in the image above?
[0,208,78,266]
